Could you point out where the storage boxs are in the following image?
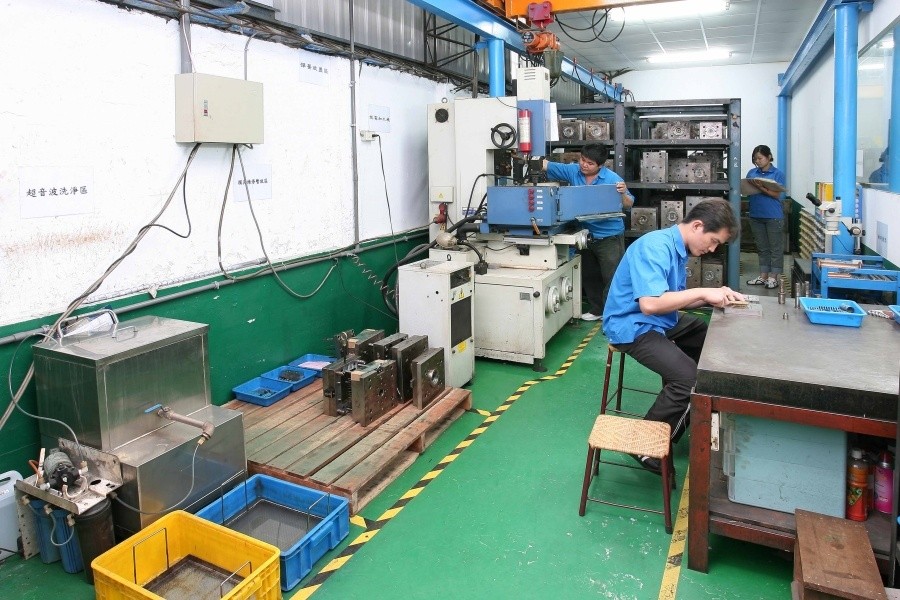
[90,509,283,600]
[194,473,351,592]
[799,297,867,327]
[718,410,847,517]
[232,354,338,407]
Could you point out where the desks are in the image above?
[687,295,900,574]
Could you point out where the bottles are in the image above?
[847,441,894,523]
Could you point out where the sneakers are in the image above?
[746,275,766,285]
[764,276,778,288]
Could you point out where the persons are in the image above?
[602,198,746,476]
[526,143,635,322]
[744,145,788,290]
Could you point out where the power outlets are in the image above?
[362,132,376,141]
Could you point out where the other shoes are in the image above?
[628,453,662,473]
[581,312,604,321]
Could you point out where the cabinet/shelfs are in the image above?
[556,98,741,289]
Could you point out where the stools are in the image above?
[600,343,666,416]
[579,414,676,533]
